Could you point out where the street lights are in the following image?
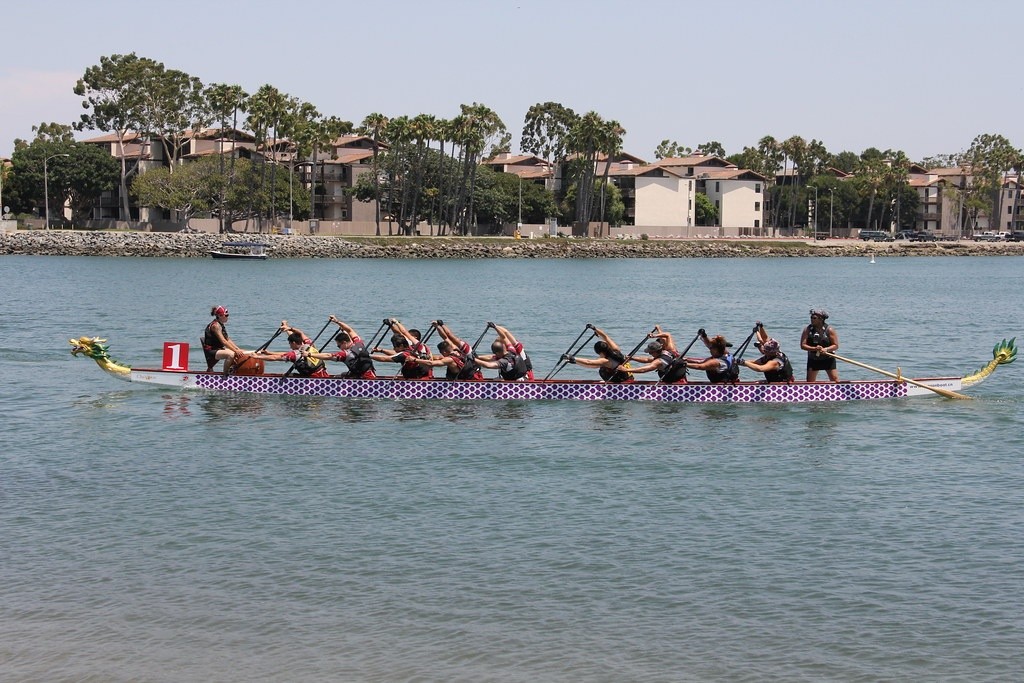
[807,186,817,243]
[828,188,833,238]
[263,161,314,228]
[519,171,526,223]
[44,153,70,230]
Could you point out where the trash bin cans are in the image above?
[308,219,320,234]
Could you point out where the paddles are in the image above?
[734,332,754,356]
[263,323,283,350]
[655,329,704,385]
[455,327,489,380]
[544,327,589,380]
[729,322,760,376]
[625,327,658,360]
[605,338,649,385]
[282,316,333,380]
[816,350,962,398]
[319,327,340,353]
[228,329,283,375]
[345,323,385,377]
[370,321,394,355]
[392,324,434,381]
[424,327,436,344]
[472,326,489,349]
[550,332,598,380]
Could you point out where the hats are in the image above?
[810,308,829,321]
[644,342,664,353]
[764,338,780,352]
[754,341,762,348]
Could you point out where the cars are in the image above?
[894,230,913,239]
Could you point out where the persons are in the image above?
[561,324,634,382]
[369,318,433,379]
[251,320,329,377]
[616,325,690,382]
[467,321,534,382]
[203,305,245,372]
[300,316,377,378]
[405,320,483,380]
[736,322,795,382]
[801,309,839,381]
[681,329,740,382]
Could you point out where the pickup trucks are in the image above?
[909,230,937,243]
[995,232,1012,242]
[971,231,995,243]
[1005,230,1024,242]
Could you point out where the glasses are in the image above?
[649,352,655,355]
[393,345,398,348]
[708,346,716,350]
[225,314,230,317]
[338,343,342,349]
[811,316,817,319]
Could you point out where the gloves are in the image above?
[737,359,745,365]
[563,353,572,360]
[756,321,764,328]
[673,359,684,367]
[753,326,758,332]
[586,324,595,330]
[698,329,707,337]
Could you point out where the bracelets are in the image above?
[414,359,416,362]
[308,353,311,358]
[395,321,398,324]
[381,349,383,353]
[289,325,292,331]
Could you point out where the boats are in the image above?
[205,242,271,260]
[69,335,1019,404]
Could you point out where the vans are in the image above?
[858,231,889,242]
